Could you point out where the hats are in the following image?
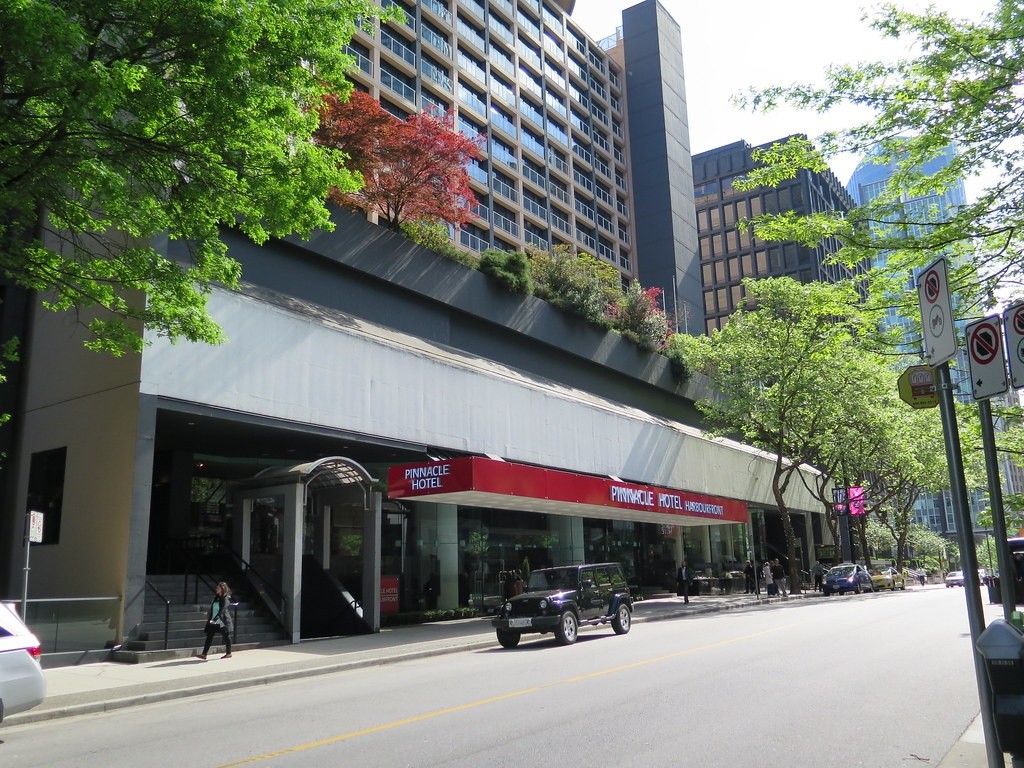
[745,560,750,563]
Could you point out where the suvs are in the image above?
[490,562,635,649]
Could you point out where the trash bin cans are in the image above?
[988,577,1002,604]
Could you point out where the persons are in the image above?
[196,582,232,659]
[764,562,776,597]
[919,567,925,585]
[744,560,764,594]
[943,568,950,576]
[770,559,788,597]
[812,561,823,592]
[678,561,693,604]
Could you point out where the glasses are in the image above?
[219,582,225,586]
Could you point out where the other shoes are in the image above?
[773,596,776,597]
[783,595,788,597]
[195,654,207,660]
[221,654,232,659]
[768,596,772,597]
[744,592,748,594]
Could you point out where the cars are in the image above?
[0,602,46,726]
[823,564,874,597]
[944,571,964,588]
[870,566,905,592]
[978,569,988,586]
